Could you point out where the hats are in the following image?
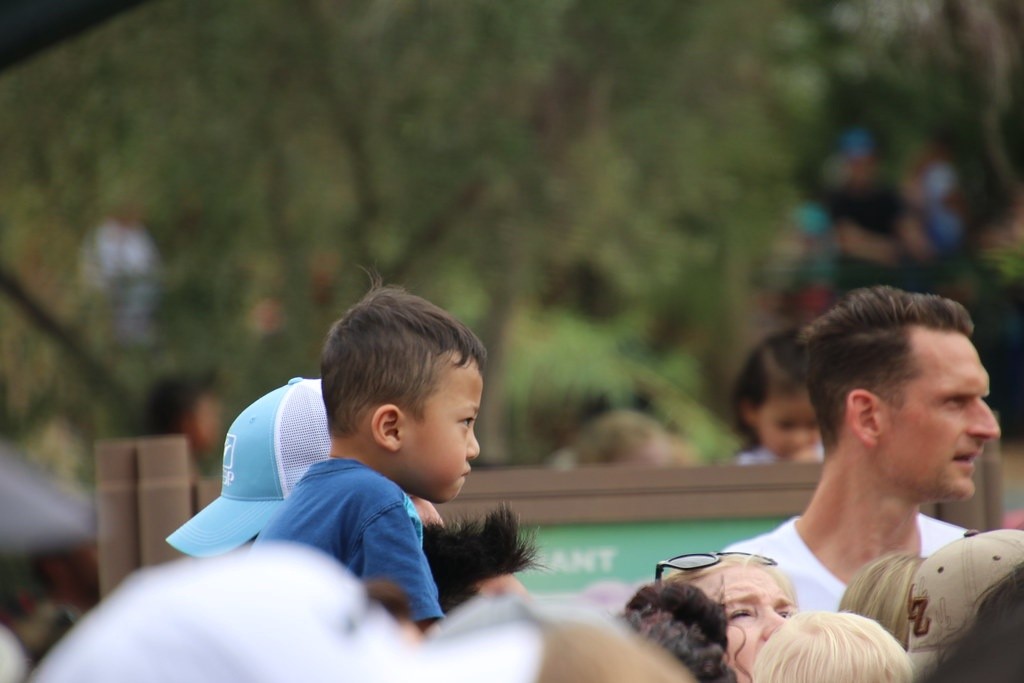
[165,377,333,559]
[25,541,543,683]
[908,529,1024,682]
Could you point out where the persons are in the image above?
[714,286,1000,616]
[0,132,1024,682]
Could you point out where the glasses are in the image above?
[657,551,778,582]
